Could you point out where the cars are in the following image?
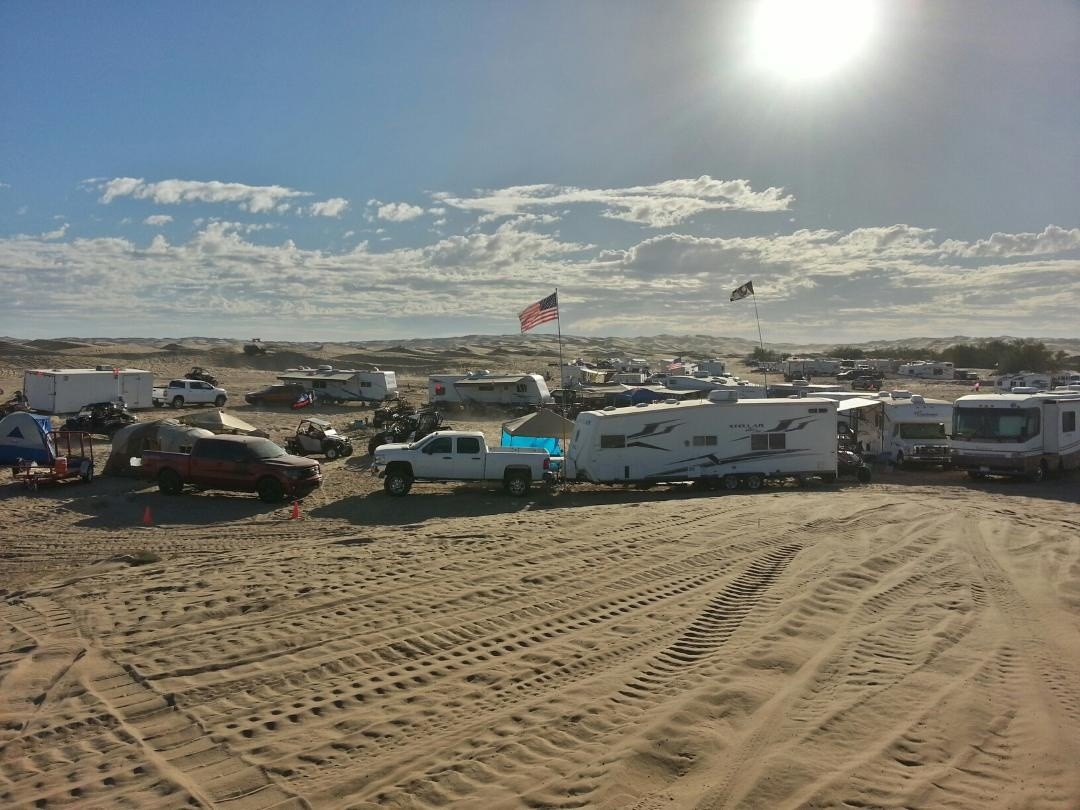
[244,381,305,410]
[284,417,354,461]
[184,366,219,387]
[837,450,871,482]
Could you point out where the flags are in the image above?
[519,293,559,332]
[730,281,754,302]
[667,358,681,370]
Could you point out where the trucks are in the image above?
[949,384,1080,484]
[853,389,955,472]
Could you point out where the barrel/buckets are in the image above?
[355,420,363,428]
[55,457,67,474]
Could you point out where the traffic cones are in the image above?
[289,501,306,521]
[143,505,153,525]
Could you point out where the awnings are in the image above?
[837,398,882,412]
[277,372,358,382]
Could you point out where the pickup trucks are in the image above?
[836,368,874,381]
[850,376,883,392]
[152,378,229,410]
[139,433,324,507]
[370,429,552,500]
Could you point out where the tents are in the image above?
[104,410,268,478]
[614,388,663,405]
[499,409,575,457]
[0,412,55,466]
